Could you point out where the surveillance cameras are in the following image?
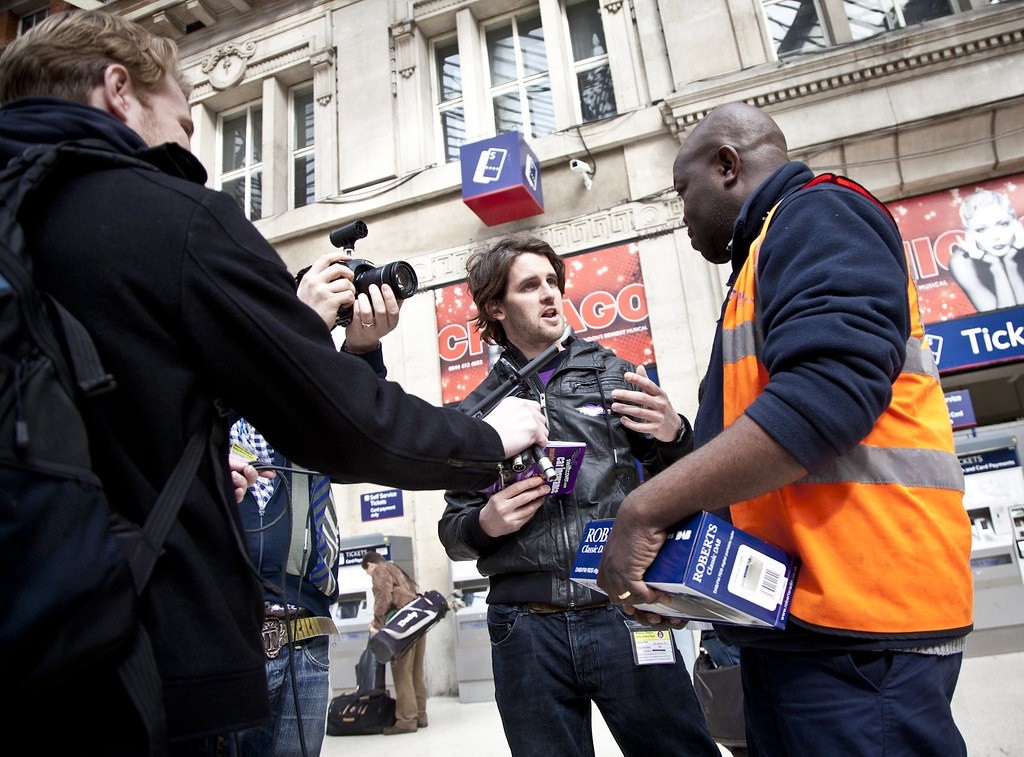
[569,159,591,173]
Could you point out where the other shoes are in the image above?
[383,724,416,735]
[416,722,428,728]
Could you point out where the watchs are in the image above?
[671,420,686,443]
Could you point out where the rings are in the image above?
[617,591,631,600]
[361,321,375,328]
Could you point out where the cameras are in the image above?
[295,220,418,328]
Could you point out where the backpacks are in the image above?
[0,136,234,755]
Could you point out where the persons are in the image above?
[362,553,428,735]
[0,11,549,757]
[949,190,1024,313]
[597,101,974,757]
[438,234,722,757]
[227,252,402,757]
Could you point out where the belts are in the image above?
[255,615,341,659]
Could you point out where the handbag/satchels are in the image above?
[326,687,396,736]
[692,652,764,748]
[356,638,386,695]
[369,588,449,664]
